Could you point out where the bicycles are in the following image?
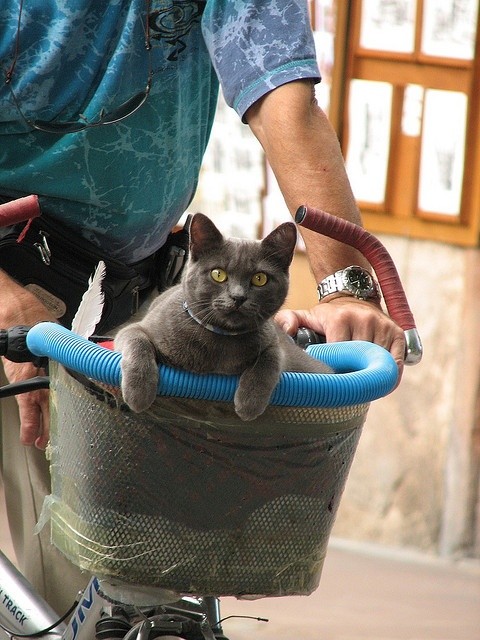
[0,194,420,640]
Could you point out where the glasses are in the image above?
[4,13,153,134]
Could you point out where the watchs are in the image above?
[316,264,382,302]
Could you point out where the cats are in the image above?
[113,213,336,421]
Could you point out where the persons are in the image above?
[0,1,407,640]
